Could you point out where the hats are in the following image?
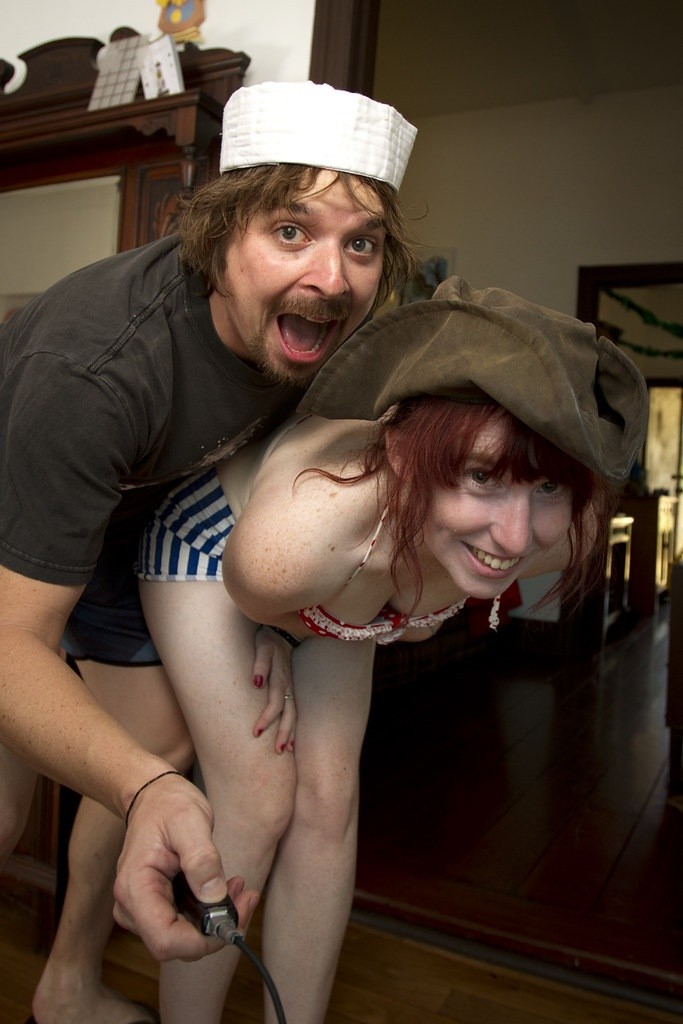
[294,276,651,479]
[220,82,418,196]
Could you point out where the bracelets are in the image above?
[127,772,181,830]
[261,621,303,649]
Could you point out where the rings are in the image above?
[284,695,295,699]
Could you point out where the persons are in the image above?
[0,78,419,1024]
[137,277,650,1024]
